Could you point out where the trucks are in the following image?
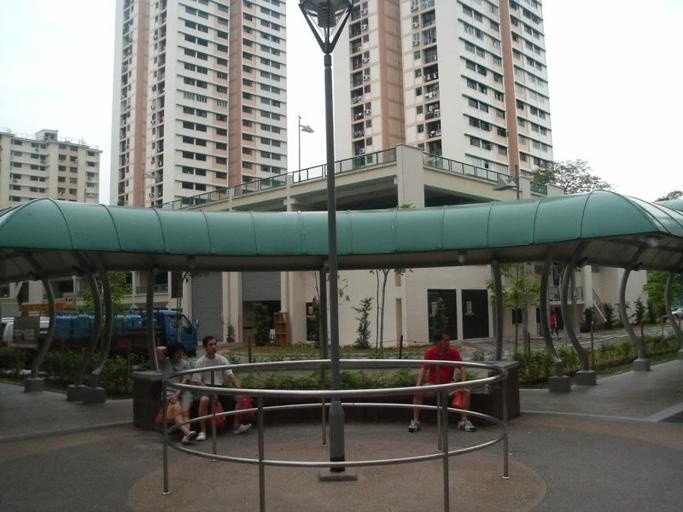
[52,308,200,363]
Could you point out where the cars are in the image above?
[672,305,683,319]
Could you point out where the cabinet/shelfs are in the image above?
[273,311,288,345]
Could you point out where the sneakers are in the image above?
[180,430,207,444]
[457,419,476,432]
[233,423,252,434]
[408,419,423,432]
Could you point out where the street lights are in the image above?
[296,0,359,472]
[296,115,314,183]
[491,164,530,353]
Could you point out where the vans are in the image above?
[0,318,51,347]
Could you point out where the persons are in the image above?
[548,310,561,340]
[191,335,253,441]
[156,340,199,446]
[407,330,478,433]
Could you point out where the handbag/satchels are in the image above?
[451,391,464,409]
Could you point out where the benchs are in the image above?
[130,360,520,432]
